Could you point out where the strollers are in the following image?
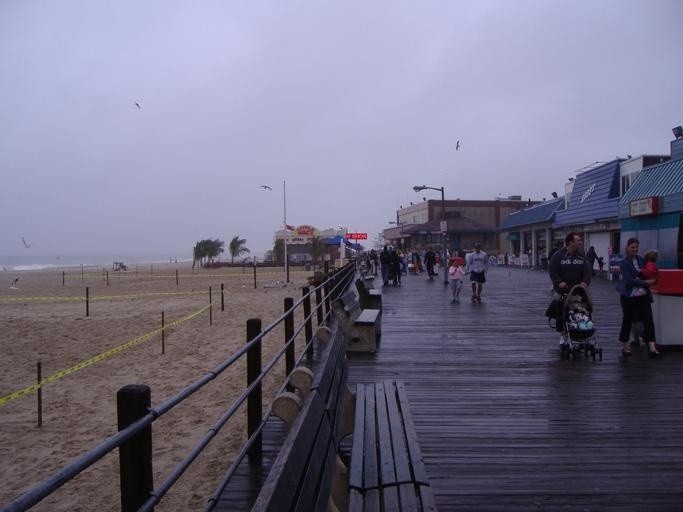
[562,285,602,361]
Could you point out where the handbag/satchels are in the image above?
[545,295,561,329]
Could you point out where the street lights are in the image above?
[413,184,448,284]
[389,221,404,250]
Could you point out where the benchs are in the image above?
[329,289,383,356]
[354,277,385,313]
[247,317,439,512]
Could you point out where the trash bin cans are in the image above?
[649,269,683,346]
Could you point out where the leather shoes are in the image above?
[648,350,662,359]
[621,349,633,356]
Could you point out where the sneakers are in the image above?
[450,298,483,305]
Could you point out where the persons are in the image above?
[448,260,466,302]
[548,231,606,345]
[356,245,467,287]
[619,237,664,360]
[465,240,489,303]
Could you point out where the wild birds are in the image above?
[455,140,460,151]
[21,237,32,250]
[134,100,141,110]
[257,185,272,190]
